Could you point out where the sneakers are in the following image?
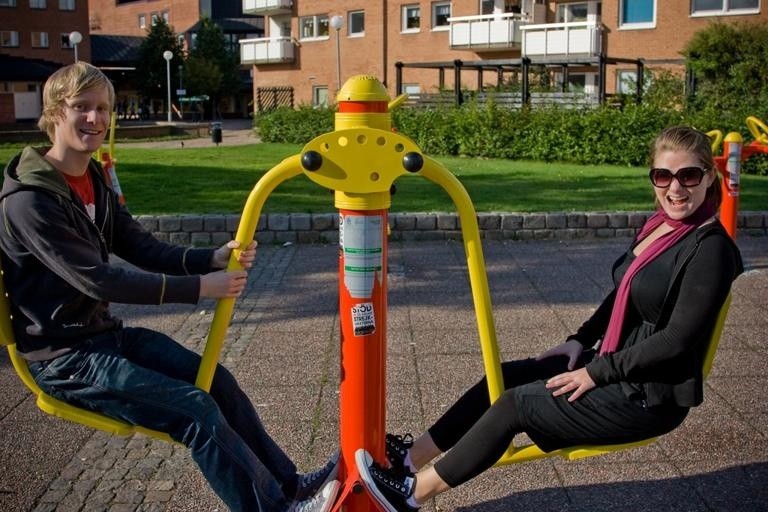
[298,448,341,501]
[291,480,340,512]
[385,431,417,477]
[355,449,421,512]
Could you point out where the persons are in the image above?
[0,62,342,511]
[353,125,747,511]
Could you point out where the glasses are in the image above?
[649,168,710,187]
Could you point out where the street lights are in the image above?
[163,51,173,121]
[330,16,344,90]
[70,32,82,63]
